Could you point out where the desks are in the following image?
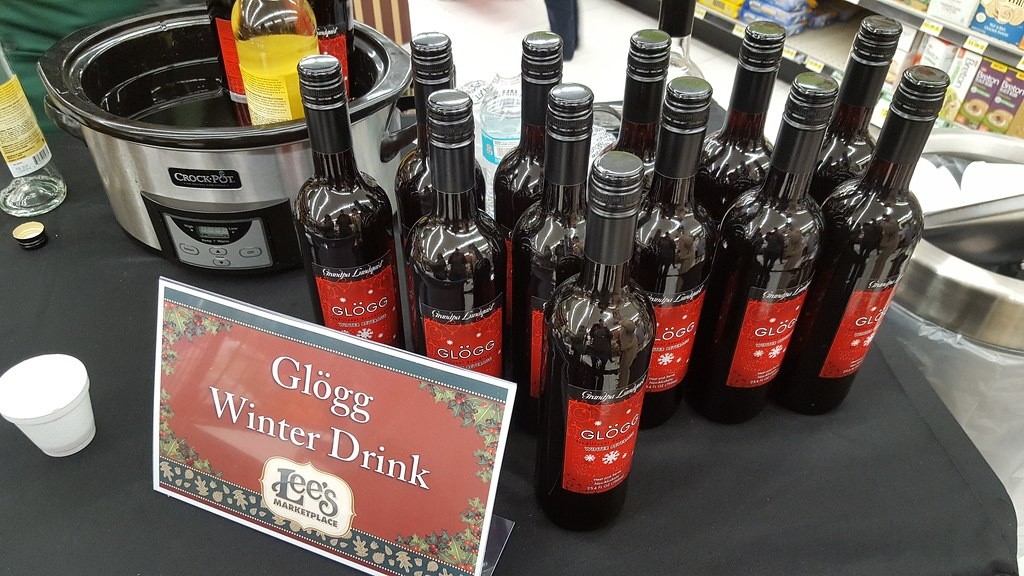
[1,93,1017,576]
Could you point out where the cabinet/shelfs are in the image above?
[615,0,1023,154]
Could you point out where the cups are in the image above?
[0,351,99,460]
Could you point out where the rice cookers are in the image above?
[46,0,416,271]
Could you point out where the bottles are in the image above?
[201,0,953,525]
[0,35,68,219]
